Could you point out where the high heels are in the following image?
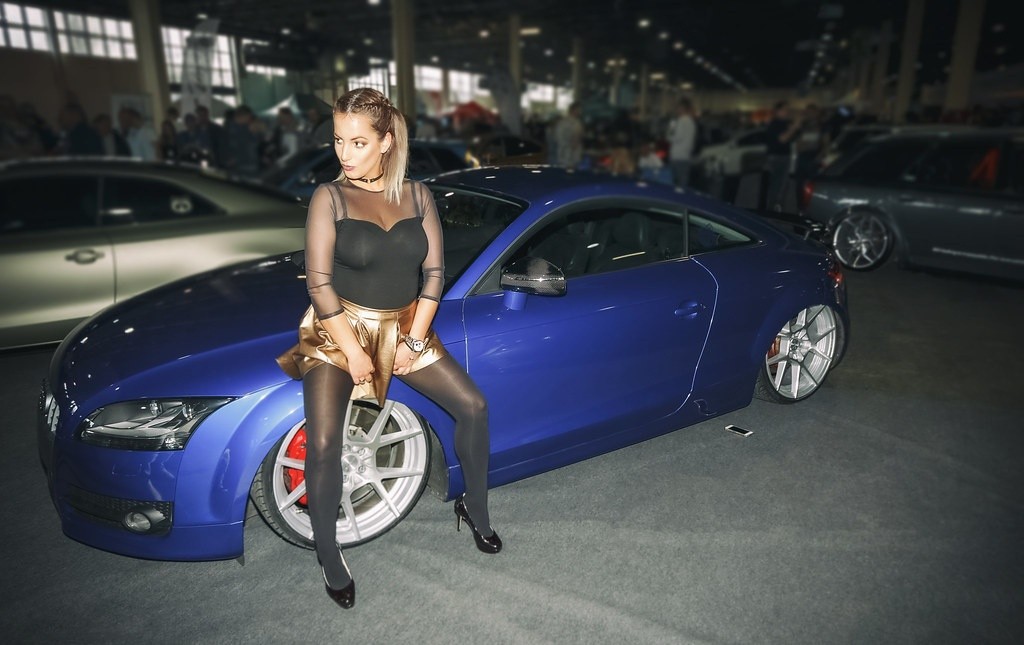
[317,542,355,609]
[454,493,502,553]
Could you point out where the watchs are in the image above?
[404,334,424,353]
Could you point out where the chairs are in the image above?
[589,210,659,277]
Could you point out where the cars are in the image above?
[805,123,1024,280]
[268,137,504,210]
[0,160,307,353]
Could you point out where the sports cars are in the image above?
[39,167,851,563]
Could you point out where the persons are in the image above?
[275,88,503,609]
[550,96,851,215]
[0,96,489,184]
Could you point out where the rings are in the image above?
[358,379,365,382]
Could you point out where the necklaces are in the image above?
[359,172,384,184]
